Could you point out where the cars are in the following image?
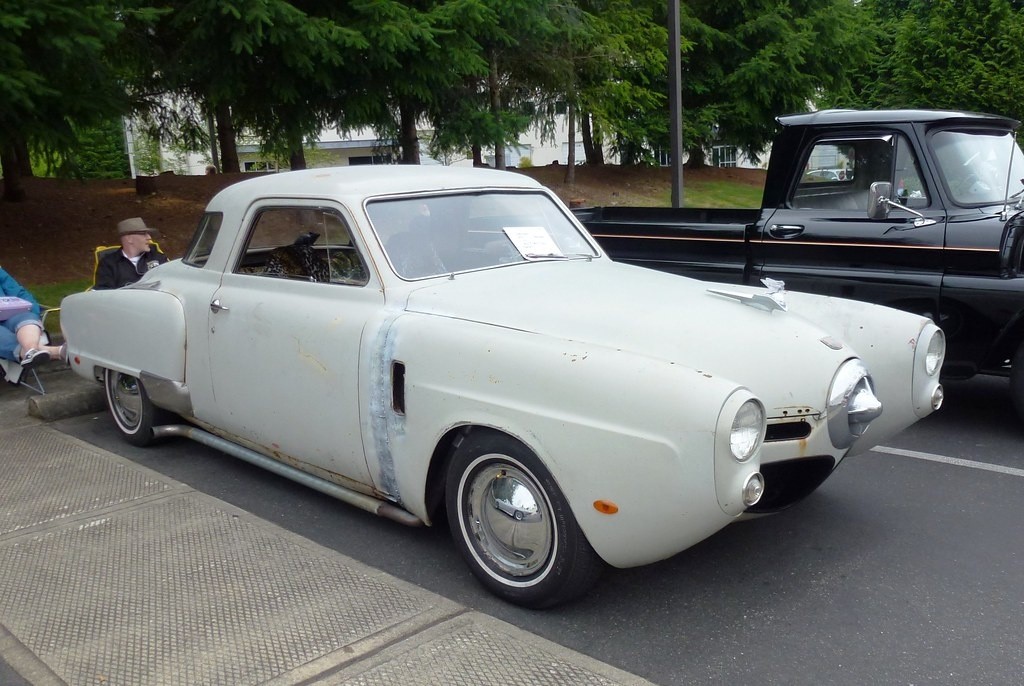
[806,170,851,181]
[58,162,948,608]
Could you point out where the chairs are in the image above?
[268,233,329,283]
[83,240,170,294]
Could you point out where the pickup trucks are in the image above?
[463,108,1023,388]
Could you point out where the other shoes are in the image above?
[60,340,71,366]
[20,348,50,368]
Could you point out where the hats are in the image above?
[118,218,156,234]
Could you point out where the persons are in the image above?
[93,217,168,291]
[0,265,70,369]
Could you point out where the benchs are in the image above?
[793,189,868,212]
